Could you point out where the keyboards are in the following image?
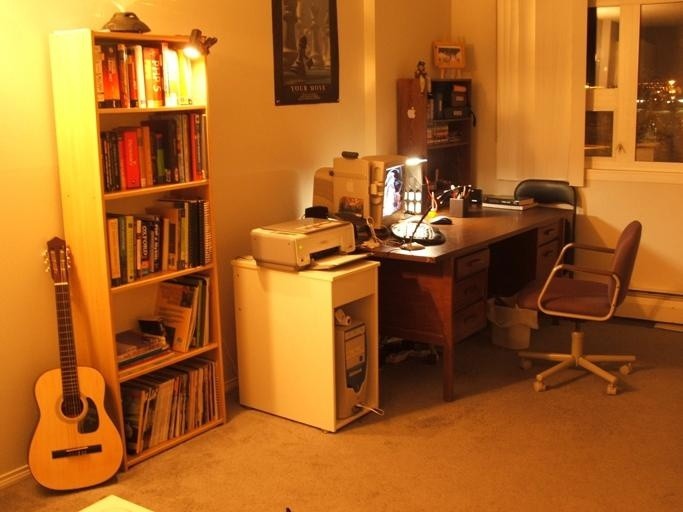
[391,220,446,244]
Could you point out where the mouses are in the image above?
[430,216,451,224]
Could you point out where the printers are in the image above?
[249,216,374,272]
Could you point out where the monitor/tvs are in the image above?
[314,155,406,240]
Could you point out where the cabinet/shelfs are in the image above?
[47,27,228,471]
[230,256,382,433]
[396,79,472,185]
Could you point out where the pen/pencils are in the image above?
[450,184,472,199]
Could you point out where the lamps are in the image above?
[183,29,218,59]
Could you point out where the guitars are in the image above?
[27,235,124,491]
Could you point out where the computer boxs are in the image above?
[336,318,367,420]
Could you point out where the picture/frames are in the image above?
[271,0,340,106]
[433,41,465,69]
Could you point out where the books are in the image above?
[101,109,207,194]
[481,197,538,211]
[106,196,213,288]
[121,358,220,458]
[95,42,193,108]
[115,272,210,364]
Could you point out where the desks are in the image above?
[364,207,573,402]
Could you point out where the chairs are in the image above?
[514,179,575,278]
[517,220,642,395]
